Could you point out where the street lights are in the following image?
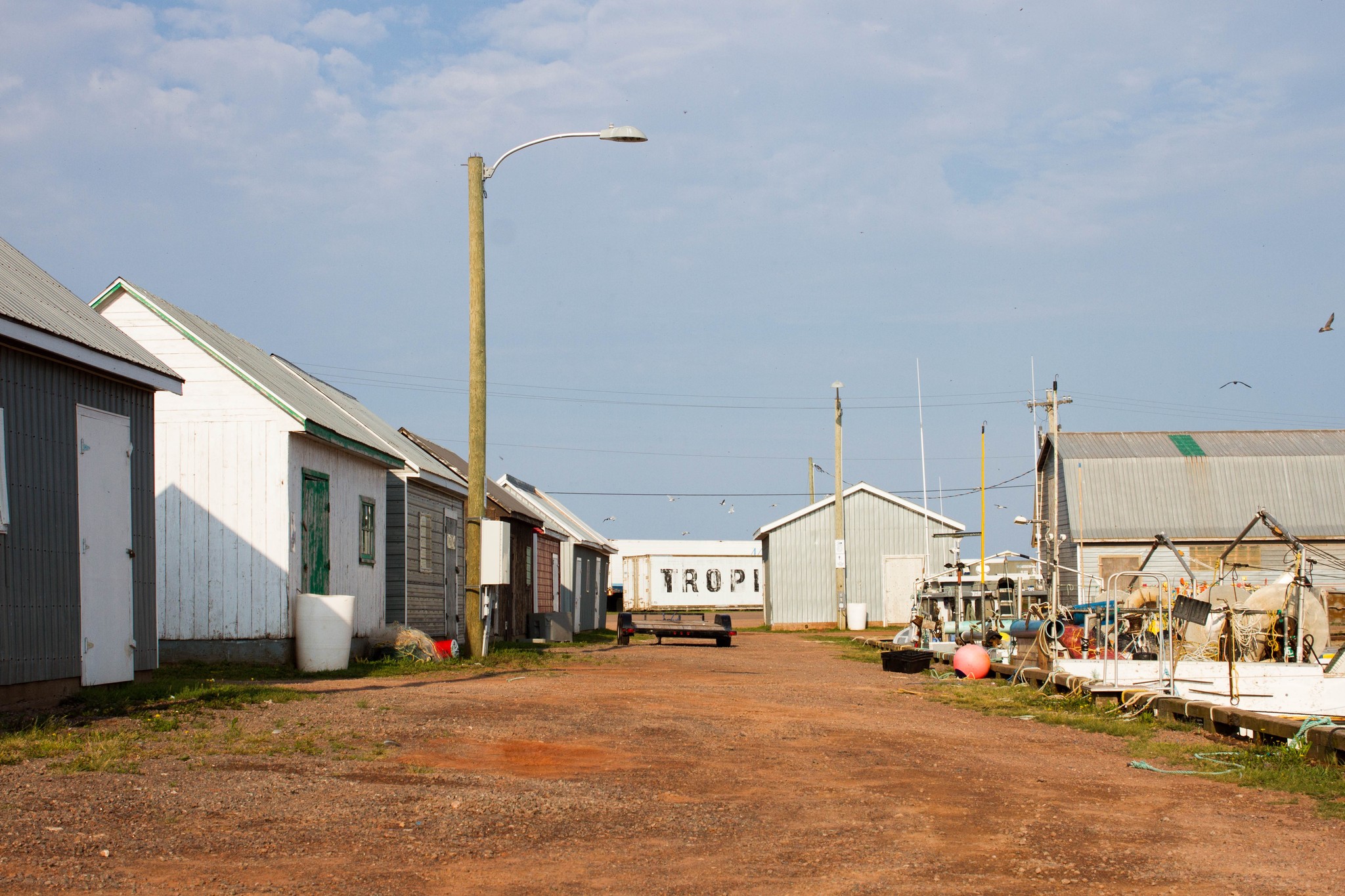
[464,123,648,660]
[1013,516,1053,618]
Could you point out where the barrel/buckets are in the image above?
[847,603,867,630]
[296,593,357,674]
[432,640,459,658]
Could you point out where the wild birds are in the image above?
[682,532,690,535]
[719,499,725,506]
[666,494,679,501]
[1319,313,1336,333]
[994,504,1007,509]
[728,504,735,515]
[499,456,503,460]
[603,518,610,523]
[1219,381,1251,388]
[769,503,779,507]
[973,487,981,491]
[610,515,616,521]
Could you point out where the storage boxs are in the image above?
[608,540,762,611]
[881,650,934,674]
